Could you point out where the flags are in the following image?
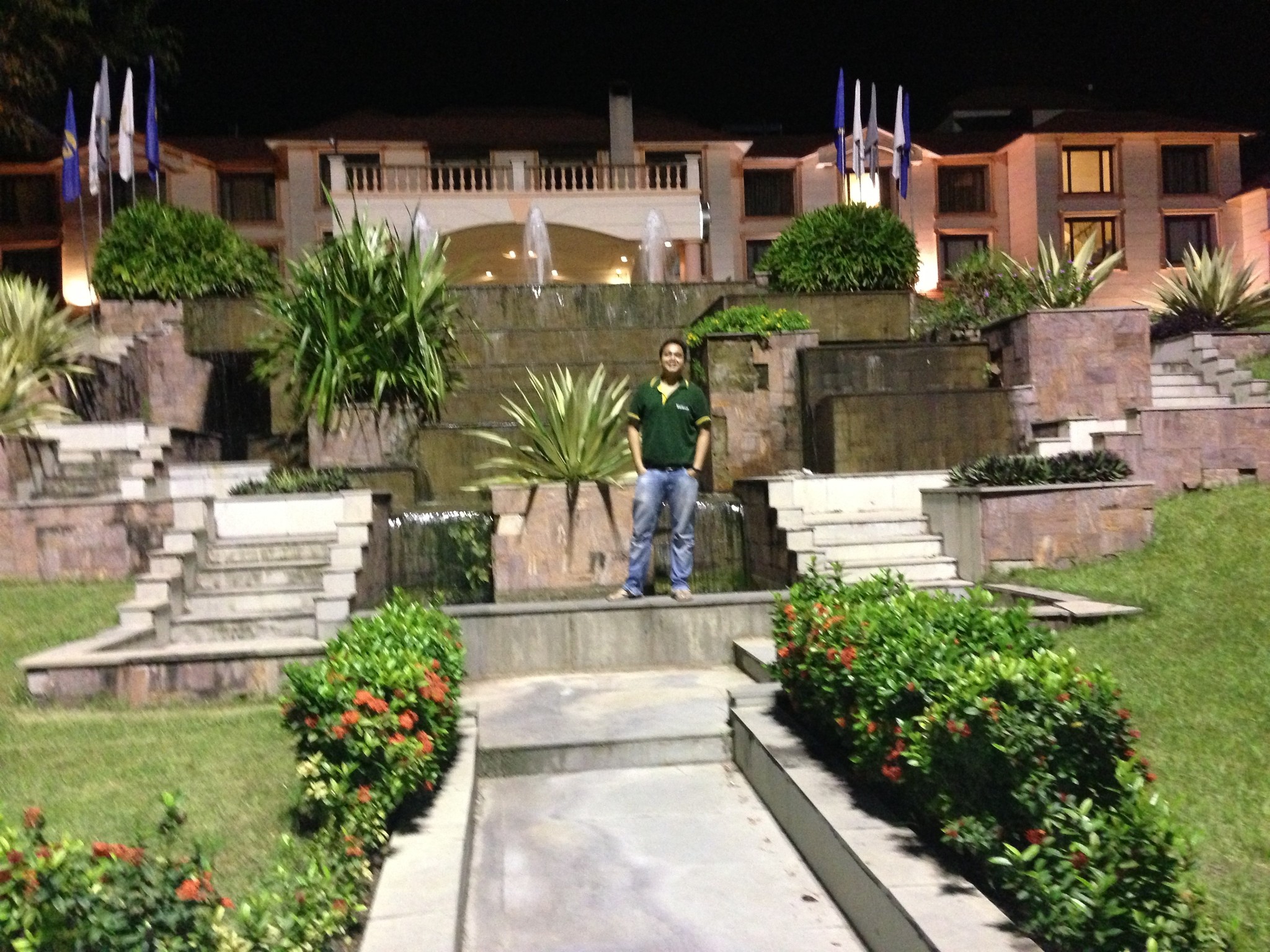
[864,83,883,187]
[61,90,82,202]
[834,68,848,177]
[145,54,160,181]
[118,67,135,182]
[95,56,111,172]
[89,81,101,196]
[892,84,912,200]
[851,80,865,179]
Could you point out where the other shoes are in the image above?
[606,586,636,602]
[668,587,693,602]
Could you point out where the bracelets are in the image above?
[691,466,702,474]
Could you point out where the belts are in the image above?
[655,465,684,472]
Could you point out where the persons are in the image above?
[605,338,712,601]
